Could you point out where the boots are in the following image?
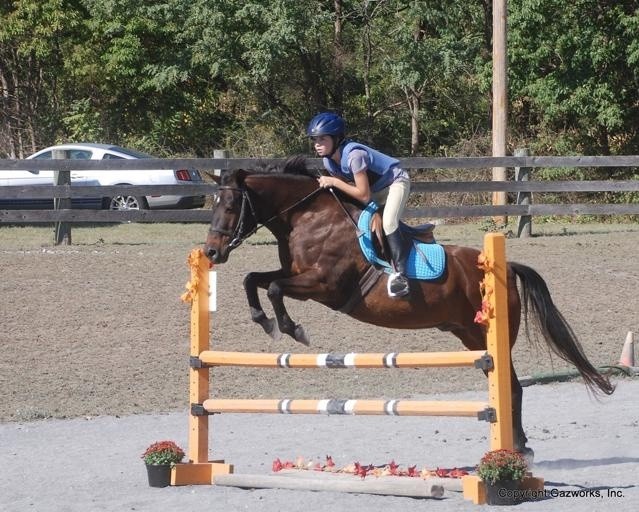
[385,225,409,294]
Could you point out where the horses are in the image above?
[200,152,617,470]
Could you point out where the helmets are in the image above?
[305,113,345,146]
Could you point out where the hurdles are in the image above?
[170,233,544,504]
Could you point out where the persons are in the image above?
[302,112,411,293]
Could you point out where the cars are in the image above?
[0,142,206,210]
